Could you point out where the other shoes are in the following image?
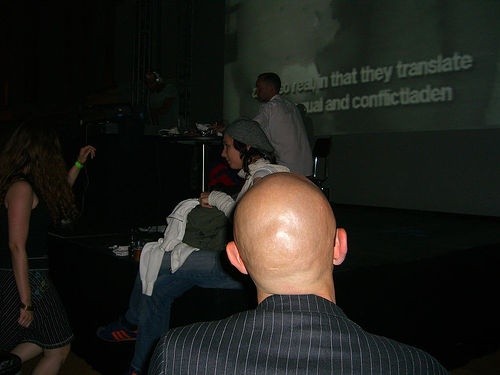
[0,353,23,375]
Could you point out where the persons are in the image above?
[97,119,293,375]
[0,118,97,375]
[210,72,315,178]
[150,173,452,375]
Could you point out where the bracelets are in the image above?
[20,301,34,312]
[74,161,84,169]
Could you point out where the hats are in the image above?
[226,120,274,152]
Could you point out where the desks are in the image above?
[172,134,222,193]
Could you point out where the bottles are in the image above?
[128,240,146,262]
[117,108,125,124]
[104,121,112,134]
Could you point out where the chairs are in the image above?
[307,138,331,193]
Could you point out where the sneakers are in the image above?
[97,311,138,342]
